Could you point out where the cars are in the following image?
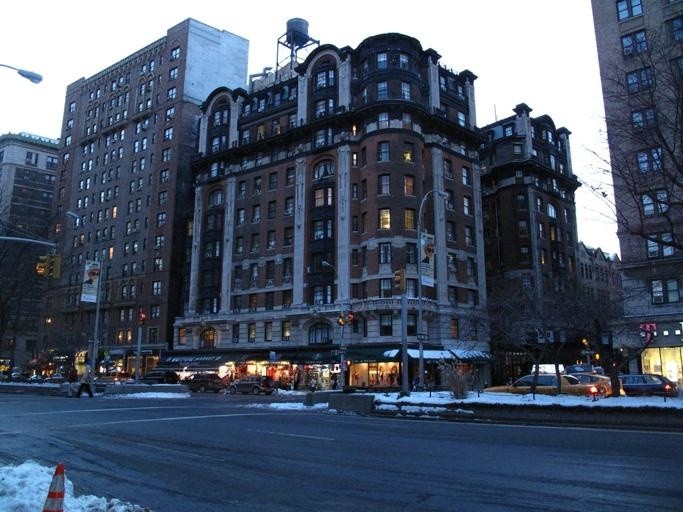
[0,369,179,391]
[483,364,678,399]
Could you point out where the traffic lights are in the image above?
[394,266,406,292]
[48,254,62,280]
[138,312,145,325]
[36,255,49,280]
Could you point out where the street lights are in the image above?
[322,261,346,387]
[65,211,104,393]
[579,336,598,364]
[416,189,451,392]
[0,63,43,84]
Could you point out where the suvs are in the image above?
[179,372,224,394]
[229,374,275,395]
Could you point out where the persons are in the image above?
[421,240,435,264]
[66,363,78,397]
[84,266,100,285]
[75,362,94,400]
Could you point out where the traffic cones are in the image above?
[42,463,67,512]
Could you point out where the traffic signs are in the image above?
[417,334,429,340]
